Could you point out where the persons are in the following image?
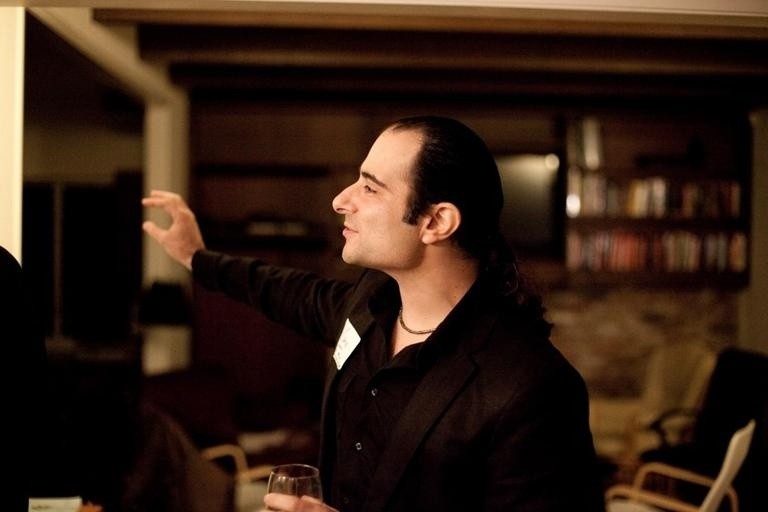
[141,109,609,511]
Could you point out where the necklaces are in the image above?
[399,305,436,335]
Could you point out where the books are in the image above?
[568,117,747,272]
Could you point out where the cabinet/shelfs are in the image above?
[554,115,750,293]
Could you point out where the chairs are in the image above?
[601,420,756,511]
[140,404,282,511]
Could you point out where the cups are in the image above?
[263,462,325,504]
